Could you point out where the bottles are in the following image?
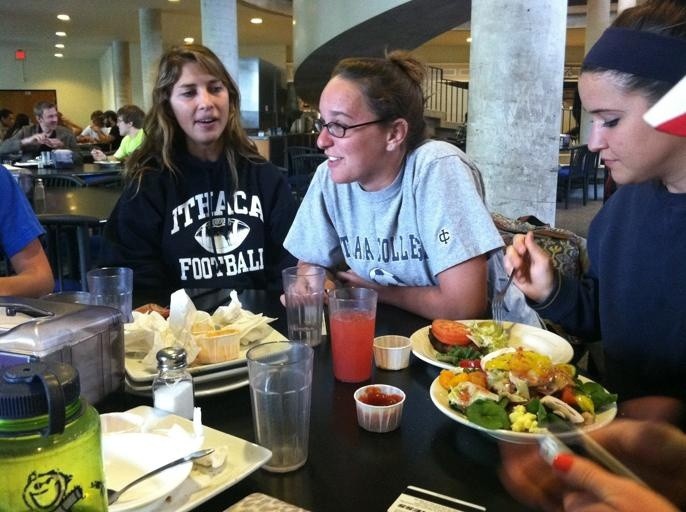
[38,155,43,168]
[151,348,195,421]
[33,179,46,198]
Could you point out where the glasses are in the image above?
[314,120,384,137]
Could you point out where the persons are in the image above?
[497,392,686,512]
[501,2,684,437]
[279,49,549,330]
[453,112,468,145]
[106,43,301,292]
[1,101,146,170]
[0,164,57,297]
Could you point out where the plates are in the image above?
[1,159,38,170]
[124,322,297,396]
[104,433,195,512]
[430,369,620,440]
[123,404,271,511]
[92,160,122,168]
[410,319,576,373]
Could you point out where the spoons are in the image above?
[104,446,216,508]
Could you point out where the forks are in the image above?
[492,250,531,323]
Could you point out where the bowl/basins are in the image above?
[96,411,145,470]
[354,384,407,432]
[375,334,413,370]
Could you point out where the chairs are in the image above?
[556,144,600,210]
[275,146,325,202]
[492,213,592,364]
[34,213,99,292]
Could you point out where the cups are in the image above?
[41,151,50,166]
[38,290,90,306]
[330,288,379,386]
[246,342,313,473]
[0,356,106,511]
[86,267,136,323]
[283,264,327,349]
